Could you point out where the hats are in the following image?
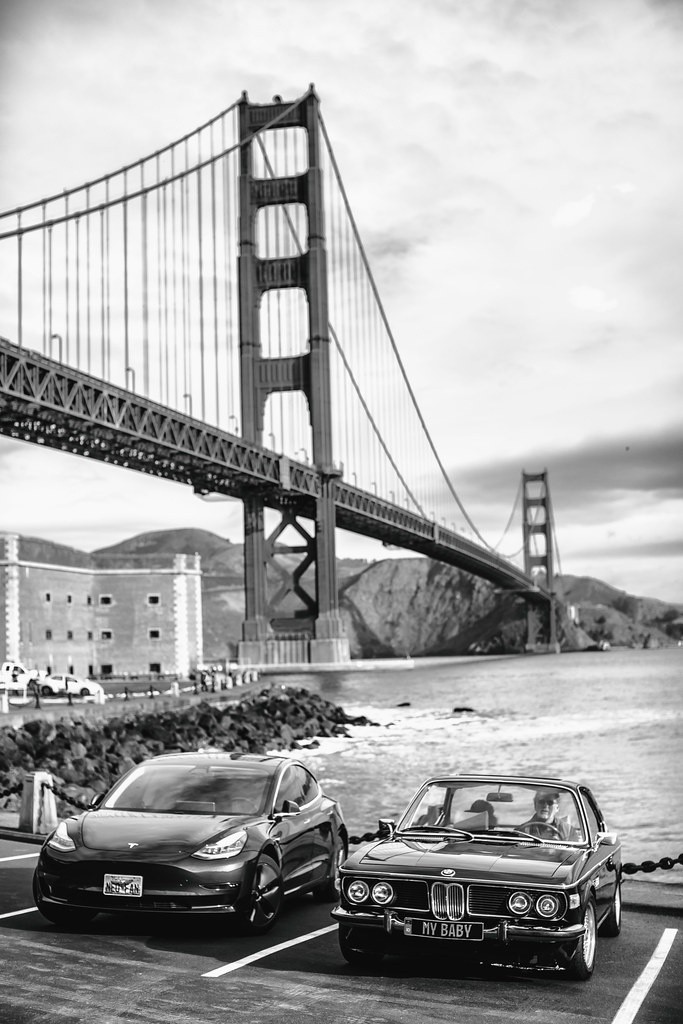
[464,800,497,826]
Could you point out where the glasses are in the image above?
[536,799,558,806]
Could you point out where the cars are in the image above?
[39,673,104,699]
[31,751,349,932]
[330,774,624,981]
[0,663,41,696]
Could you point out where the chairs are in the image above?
[559,815,570,823]
[454,810,488,832]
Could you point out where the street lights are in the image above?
[301,448,309,466]
[371,481,378,496]
[183,394,193,417]
[50,333,63,365]
[352,472,358,488]
[405,498,473,539]
[125,367,136,394]
[269,433,276,452]
[388,490,396,504]
[229,416,238,437]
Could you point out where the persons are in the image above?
[510,790,579,844]
[188,666,232,694]
